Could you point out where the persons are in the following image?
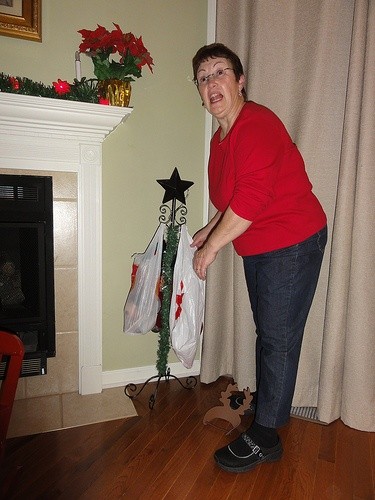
[190,43,328,472]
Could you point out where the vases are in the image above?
[96,78,131,107]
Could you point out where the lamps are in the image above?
[74,51,81,82]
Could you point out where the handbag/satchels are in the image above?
[124,222,167,335]
[169,225,205,369]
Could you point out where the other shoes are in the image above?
[215,433,282,472]
[231,393,255,413]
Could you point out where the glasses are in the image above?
[194,67,232,88]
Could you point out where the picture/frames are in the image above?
[0,0,43,42]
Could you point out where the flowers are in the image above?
[77,22,155,81]
[0,72,109,105]
